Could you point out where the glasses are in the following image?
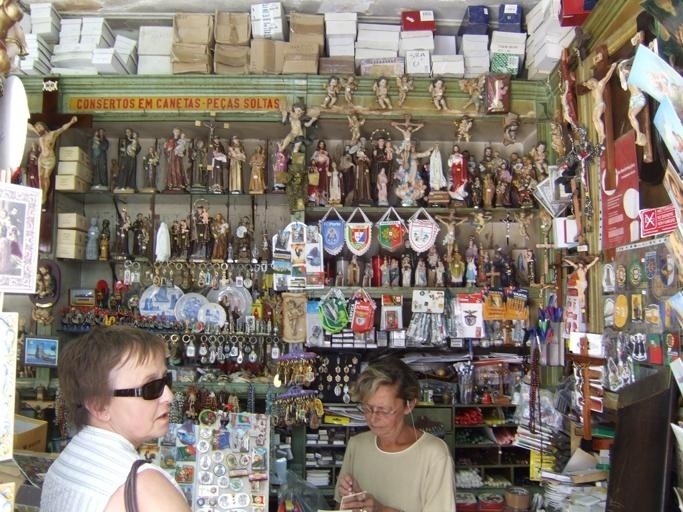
[114,372,172,400]
[356,403,405,417]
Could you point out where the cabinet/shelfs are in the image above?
[1,1,683,512]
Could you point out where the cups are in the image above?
[458,383,474,405]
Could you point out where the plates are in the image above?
[139,285,254,330]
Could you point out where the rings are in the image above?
[359,507,367,512]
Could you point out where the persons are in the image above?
[562,256,600,309]
[617,55,647,146]
[7,225,23,259]
[486,79,509,112]
[26,115,78,208]
[373,75,393,110]
[396,75,412,105]
[582,61,617,147]
[557,77,580,132]
[461,77,483,112]
[275,101,317,154]
[329,354,457,512]
[319,77,340,109]
[345,112,367,141]
[39,324,193,512]
[81,114,549,289]
[427,78,448,111]
[339,75,358,106]
[667,175,682,223]
[0,208,10,227]
[8,206,23,232]
[0,224,12,273]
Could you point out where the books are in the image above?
[513,418,575,512]
